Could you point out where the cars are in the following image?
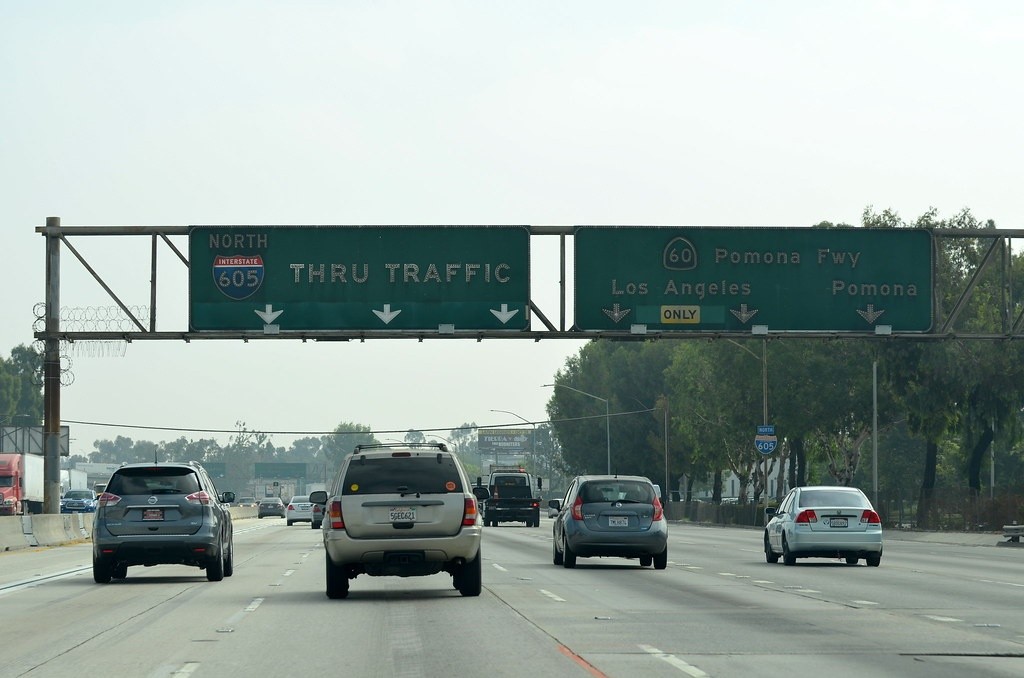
[61,488,98,513]
[254,496,287,519]
[284,495,314,526]
[217,490,232,507]
[548,473,668,570]
[94,484,107,499]
[308,490,331,529]
[763,483,885,571]
[237,497,256,508]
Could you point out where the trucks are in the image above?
[477,469,543,528]
[0,453,44,516]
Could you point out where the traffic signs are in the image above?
[573,225,937,335]
[188,224,531,332]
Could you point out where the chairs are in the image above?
[625,491,638,500]
[584,489,605,504]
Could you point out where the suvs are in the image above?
[310,442,490,602]
[87,451,236,584]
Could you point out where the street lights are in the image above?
[542,384,611,476]
[491,409,536,498]
[425,434,457,454]
